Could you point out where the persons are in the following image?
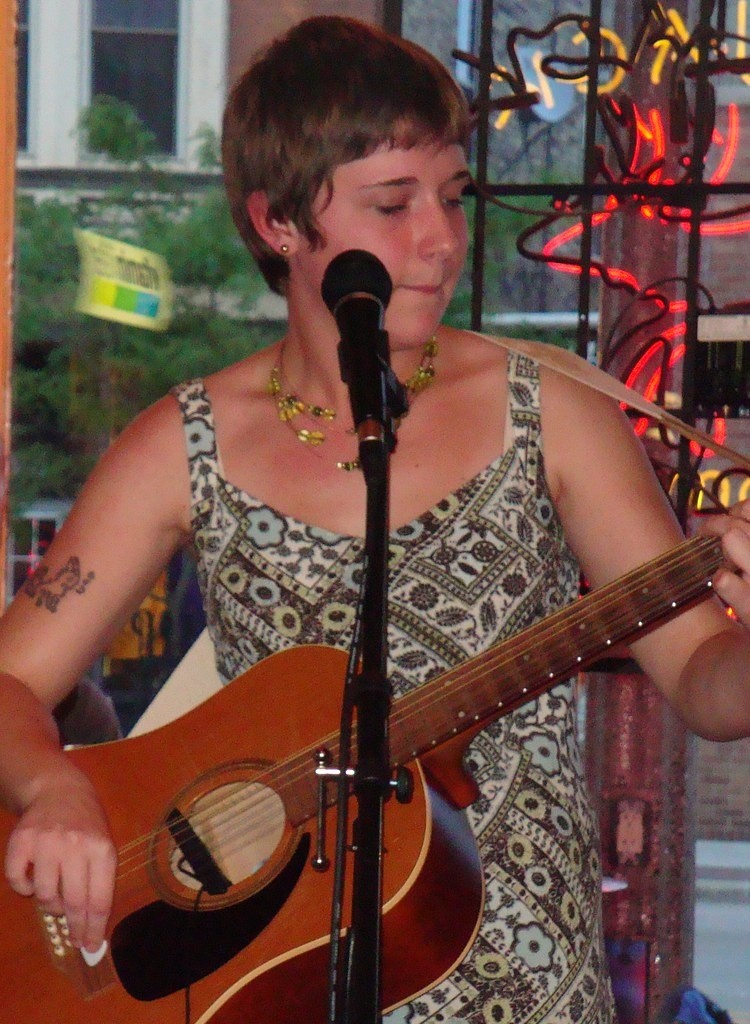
[0,15,749,1024]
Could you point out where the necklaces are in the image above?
[264,334,441,467]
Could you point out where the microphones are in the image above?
[318,248,394,485]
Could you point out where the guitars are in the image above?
[0,499,750,1024]
